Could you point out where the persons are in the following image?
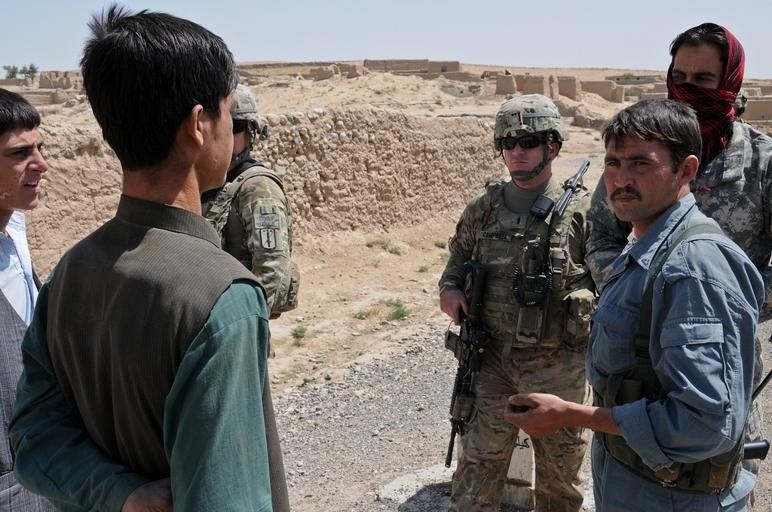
[586,22,768,506]
[438,93,589,511]
[201,82,299,359]
[0,88,53,510]
[504,97,765,509]
[8,2,290,511]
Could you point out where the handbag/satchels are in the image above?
[600,225,745,494]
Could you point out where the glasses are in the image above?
[233,120,248,133]
[500,134,547,150]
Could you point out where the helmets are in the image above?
[494,91,565,143]
[228,83,263,134]
